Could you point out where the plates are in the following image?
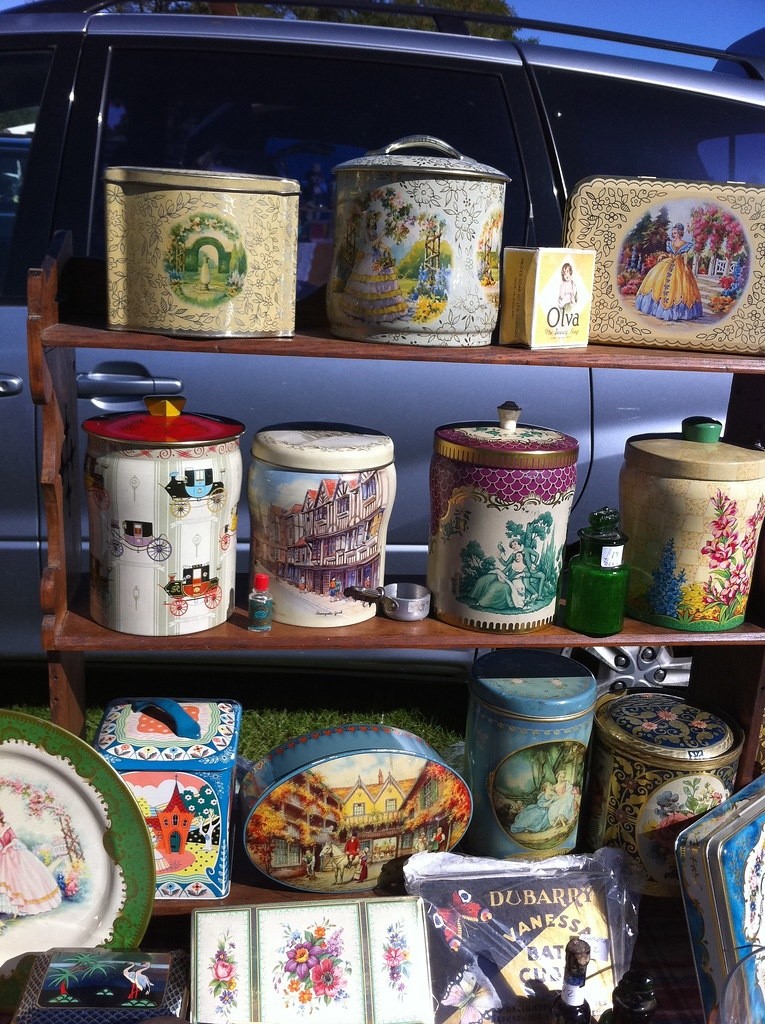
[0,708,156,1014]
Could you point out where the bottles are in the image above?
[600,969,657,1024]
[549,939,591,1024]
[247,573,272,632]
[563,508,629,636]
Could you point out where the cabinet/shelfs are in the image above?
[25,229,765,915]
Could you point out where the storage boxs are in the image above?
[498,245,597,350]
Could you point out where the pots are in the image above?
[343,582,430,622]
